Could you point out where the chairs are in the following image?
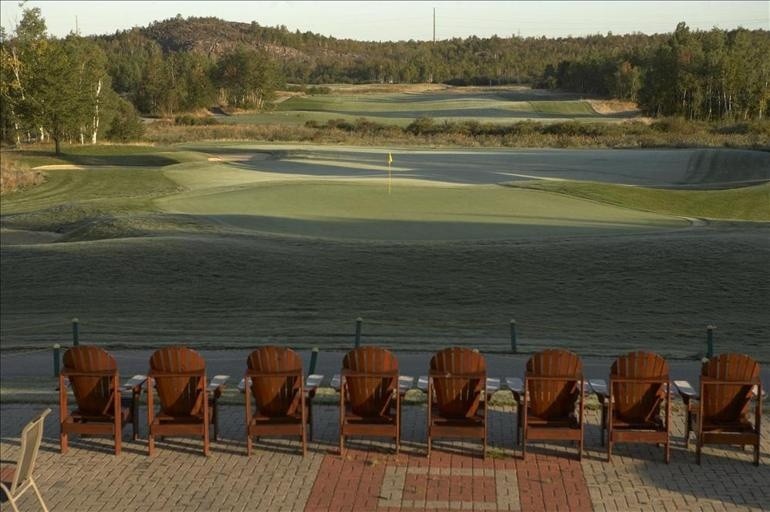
[0,408,53,512]
